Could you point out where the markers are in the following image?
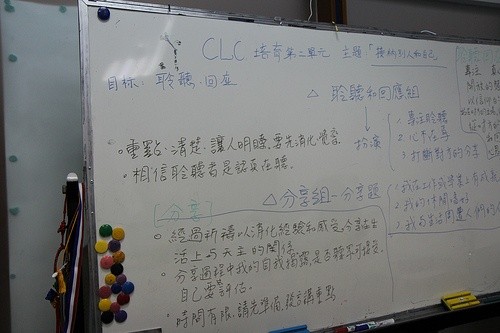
[335,320,374,332]
[355,316,401,331]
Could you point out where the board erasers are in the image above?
[441,290,480,311]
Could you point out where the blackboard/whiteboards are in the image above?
[77,0,499,333]
